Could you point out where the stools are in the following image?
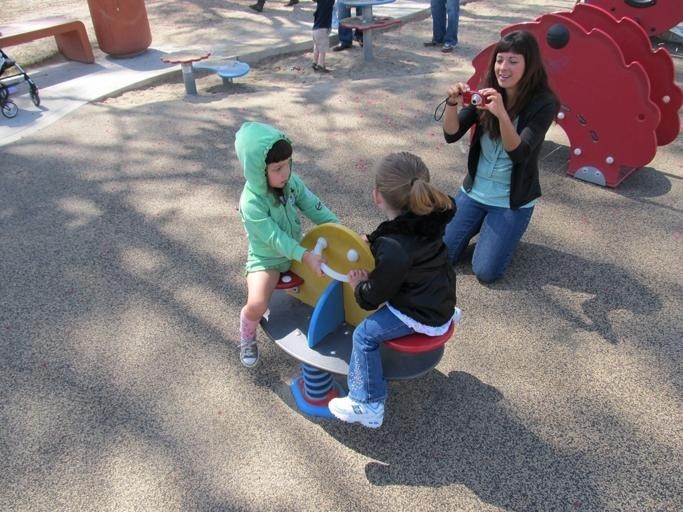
[159,47,249,94]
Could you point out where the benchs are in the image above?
[0,14,94,66]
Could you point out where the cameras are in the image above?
[462,90,486,107]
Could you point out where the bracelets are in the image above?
[445,97,458,107]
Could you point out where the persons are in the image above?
[330,0,363,52]
[441,29,561,283]
[246,0,299,13]
[326,151,458,431]
[311,0,335,73]
[234,122,344,368]
[423,0,460,53]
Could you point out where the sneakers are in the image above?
[248,4,262,12]
[240,337,258,368]
[284,0,299,6]
[424,39,455,52]
[329,396,385,428]
[333,34,363,51]
[312,63,330,73]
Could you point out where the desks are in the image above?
[337,0,399,61]
